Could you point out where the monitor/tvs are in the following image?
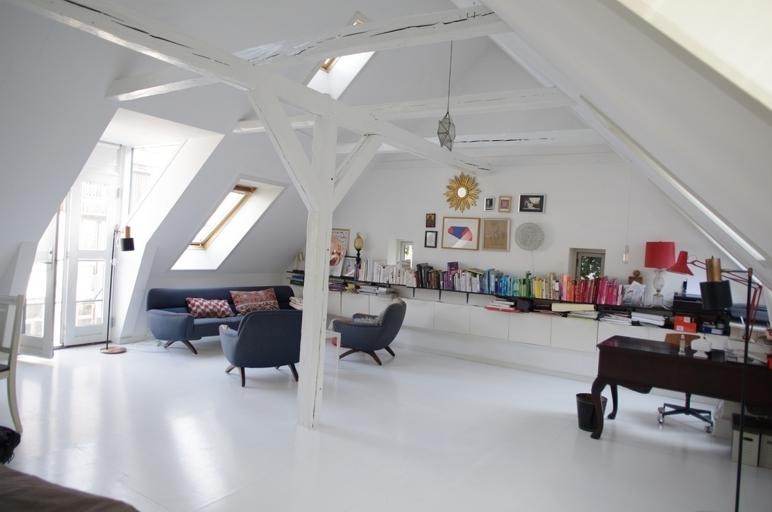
[700,280,732,310]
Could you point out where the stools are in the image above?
[657,331,716,434]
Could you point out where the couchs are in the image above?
[332,301,406,366]
[217,310,302,387]
[145,284,301,355]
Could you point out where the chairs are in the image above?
[0,296,24,440]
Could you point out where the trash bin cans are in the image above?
[731,413,758,466]
[576,392,607,432]
[758,417,771,468]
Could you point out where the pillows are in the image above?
[186,296,234,320]
[230,288,279,314]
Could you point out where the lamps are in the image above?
[355,237,363,259]
[700,255,755,511]
[99,226,135,354]
[644,240,674,306]
[667,248,763,341]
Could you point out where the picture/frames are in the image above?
[331,229,350,256]
[483,197,496,211]
[424,231,438,249]
[518,194,546,214]
[341,257,357,280]
[426,214,436,228]
[441,216,481,251]
[330,254,345,279]
[481,218,511,252]
[498,196,512,212]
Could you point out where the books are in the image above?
[359,258,625,305]
[724,339,768,366]
[485,296,520,312]
[630,311,670,327]
[551,303,598,313]
[356,285,399,298]
[598,310,632,326]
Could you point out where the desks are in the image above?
[590,334,772,441]
